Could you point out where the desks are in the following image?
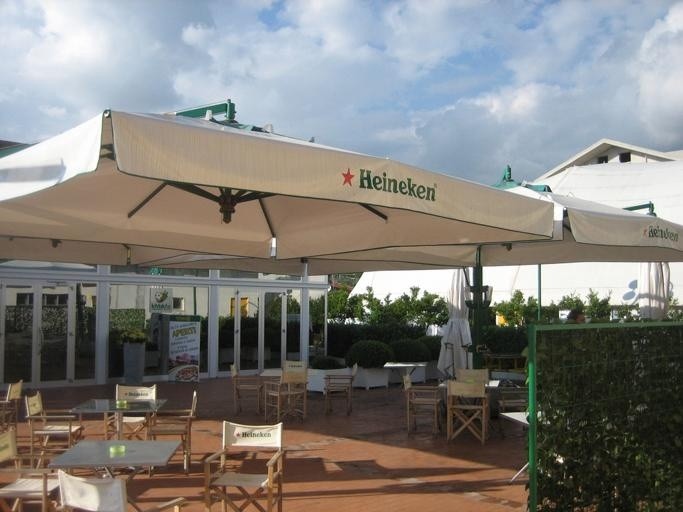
[67,398,168,440]
[383,360,429,402]
[48,438,184,512]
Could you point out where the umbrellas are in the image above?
[437,268,473,377]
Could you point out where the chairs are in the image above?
[230,365,264,411]
[402,368,492,445]
[147,390,198,476]
[24,391,83,467]
[324,365,357,416]
[57,468,186,512]
[204,419,285,512]
[1,379,24,431]
[0,427,59,512]
[266,363,308,422]
